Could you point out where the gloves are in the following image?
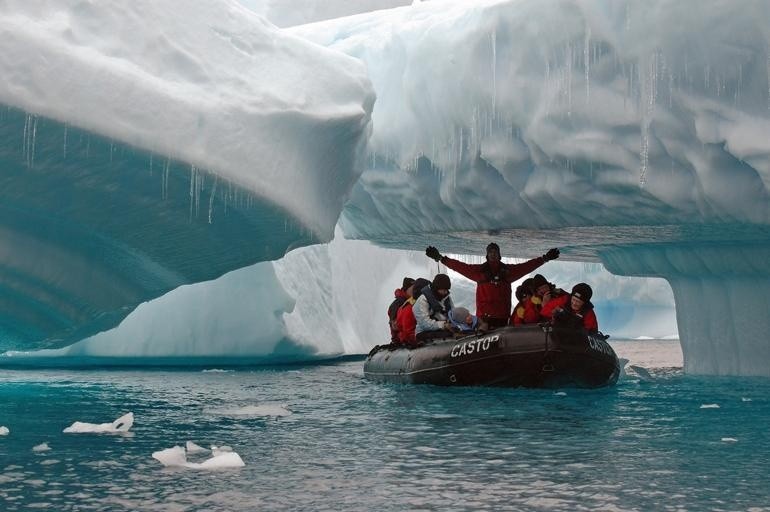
[543,247,560,262]
[426,245,441,262]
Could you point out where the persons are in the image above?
[509,274,597,332]
[388,274,488,349]
[426,242,560,327]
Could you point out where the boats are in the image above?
[363,326,621,389]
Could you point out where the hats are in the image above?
[515,273,547,300]
[486,243,501,260]
[403,274,451,299]
[571,283,592,303]
[451,307,469,323]
